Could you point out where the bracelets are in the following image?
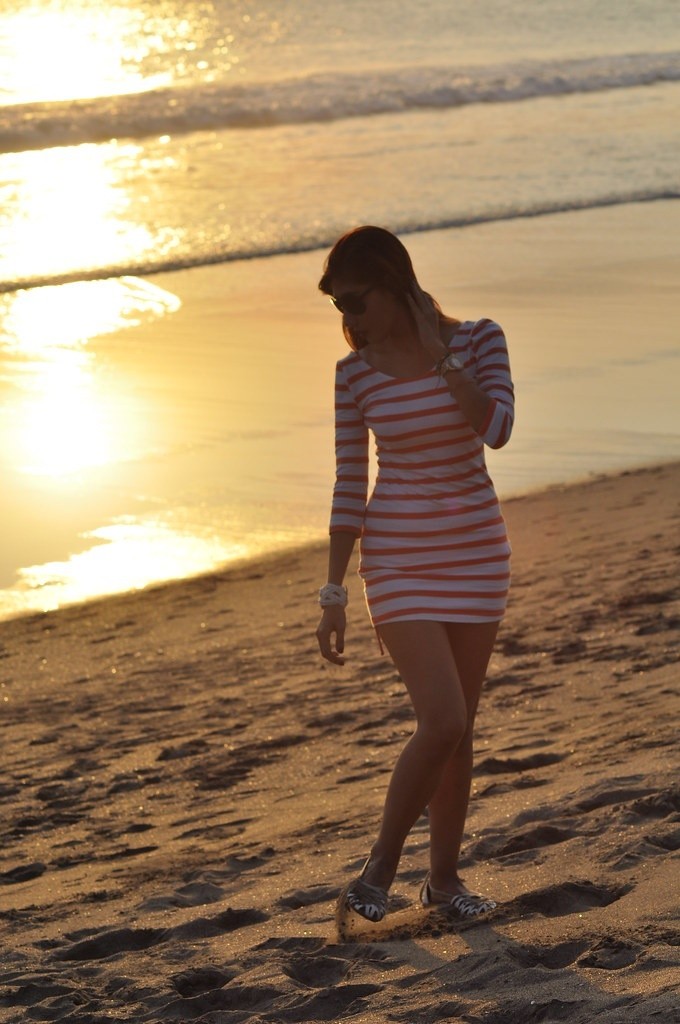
[435,351,455,369]
[318,584,348,608]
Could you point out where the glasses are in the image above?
[332,287,371,315]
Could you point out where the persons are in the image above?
[313,222,516,922]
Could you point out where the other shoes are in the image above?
[347,857,391,922]
[421,882,496,918]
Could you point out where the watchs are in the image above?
[439,352,464,377]
